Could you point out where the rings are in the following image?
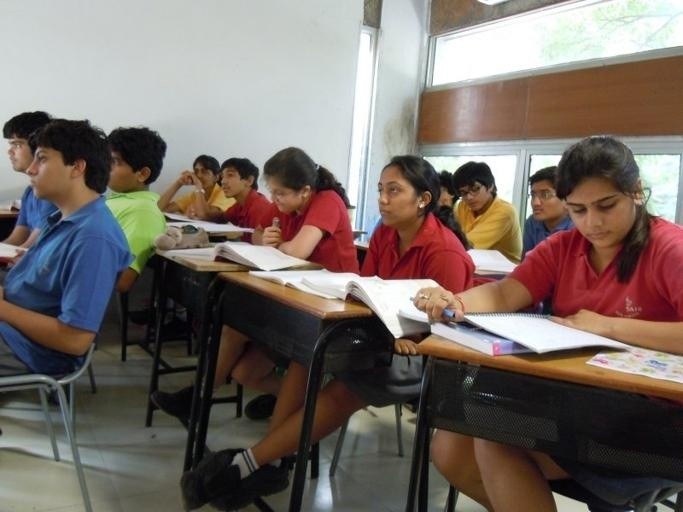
[440,295,449,302]
[419,293,430,300]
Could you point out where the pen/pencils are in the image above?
[410,296,456,319]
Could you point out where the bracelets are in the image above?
[454,295,464,312]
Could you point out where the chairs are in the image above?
[330,367,427,472]
[1,346,97,512]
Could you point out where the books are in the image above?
[0,242,29,259]
[169,241,310,271]
[429,321,535,356]
[162,213,196,222]
[467,248,518,275]
[166,222,254,233]
[246,269,341,300]
[301,276,441,339]
[0,199,25,210]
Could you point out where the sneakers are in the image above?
[243,394,276,419]
[151,384,213,422]
[179,446,244,511]
[209,465,288,509]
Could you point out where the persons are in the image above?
[158,155,238,222]
[2,113,60,267]
[148,147,365,426]
[450,162,521,265]
[0,118,135,387]
[100,127,169,295]
[411,139,681,510]
[518,164,576,262]
[179,159,477,511]
[183,158,270,243]
[431,170,459,211]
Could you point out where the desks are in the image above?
[1,201,23,232]
[188,267,401,507]
[117,221,241,362]
[405,314,681,512]
[145,244,321,431]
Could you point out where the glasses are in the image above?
[457,184,483,196]
[526,191,555,200]
